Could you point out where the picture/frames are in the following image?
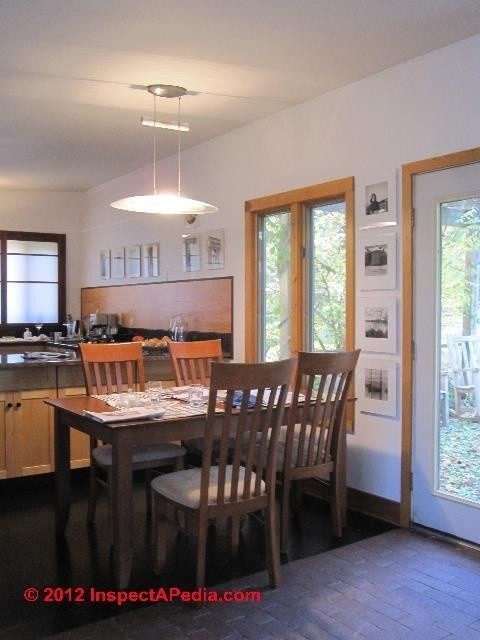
[359,297,397,356]
[357,355,398,418]
[100,227,224,279]
[356,168,398,231]
[358,234,396,290]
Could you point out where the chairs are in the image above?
[167,339,273,525]
[151,357,297,607]
[235,348,360,554]
[445,335,480,422]
[78,341,186,552]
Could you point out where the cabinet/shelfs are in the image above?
[0,389,57,480]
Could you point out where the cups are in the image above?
[54,332,62,342]
[188,384,205,407]
[148,380,162,406]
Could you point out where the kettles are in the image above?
[72,320,83,338]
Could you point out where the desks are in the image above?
[441,371,448,423]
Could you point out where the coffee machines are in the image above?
[87,313,117,340]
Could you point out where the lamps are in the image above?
[110,84,219,215]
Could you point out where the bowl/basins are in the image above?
[142,346,168,355]
